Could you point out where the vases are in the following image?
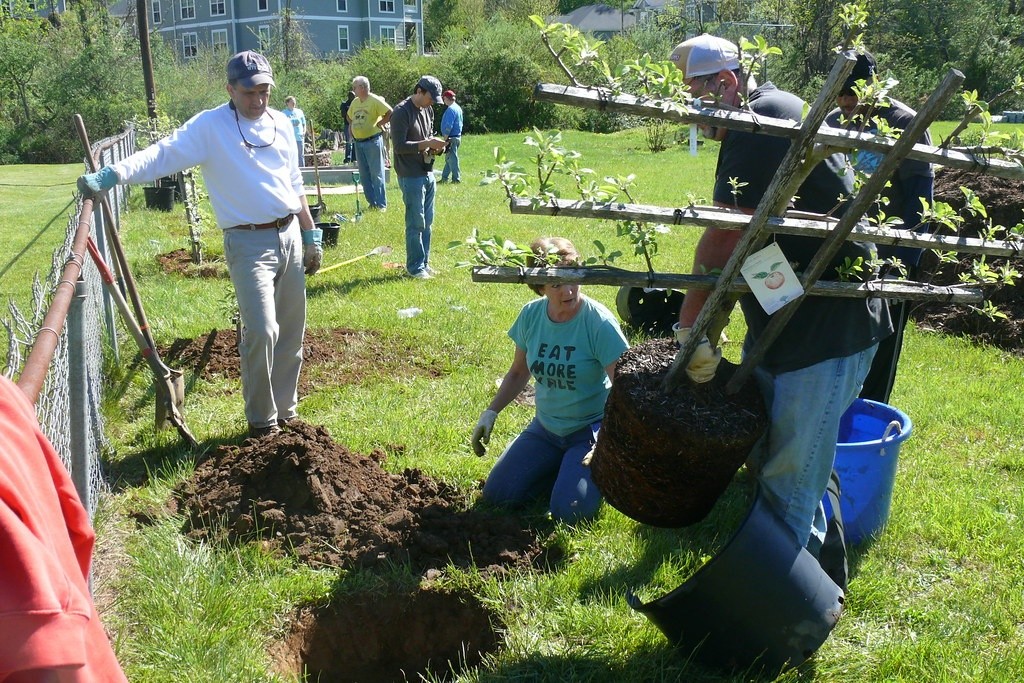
[308,205,322,223]
[315,222,340,249]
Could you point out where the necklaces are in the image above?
[233,106,277,149]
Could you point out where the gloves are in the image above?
[302,228,323,275]
[672,321,722,383]
[76,166,119,211]
[472,410,498,457]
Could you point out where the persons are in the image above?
[664,30,896,545]
[75,51,320,437]
[338,91,357,163]
[345,75,393,211]
[377,95,392,169]
[388,75,449,279]
[825,48,935,404]
[436,90,464,184]
[280,96,306,168]
[468,235,632,524]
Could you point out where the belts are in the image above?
[229,214,293,229]
[355,132,383,142]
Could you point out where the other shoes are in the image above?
[277,416,293,427]
[409,269,431,278]
[424,263,434,275]
[248,423,283,438]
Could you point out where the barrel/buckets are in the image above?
[809,397,913,548]
[312,223,340,249]
[142,185,175,213]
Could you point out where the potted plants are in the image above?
[120,98,182,213]
[156,115,182,204]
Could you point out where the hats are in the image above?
[418,75,445,104]
[832,50,877,90]
[670,32,742,79]
[444,90,456,97]
[227,49,276,87]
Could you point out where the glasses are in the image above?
[838,90,858,97]
[235,122,276,147]
